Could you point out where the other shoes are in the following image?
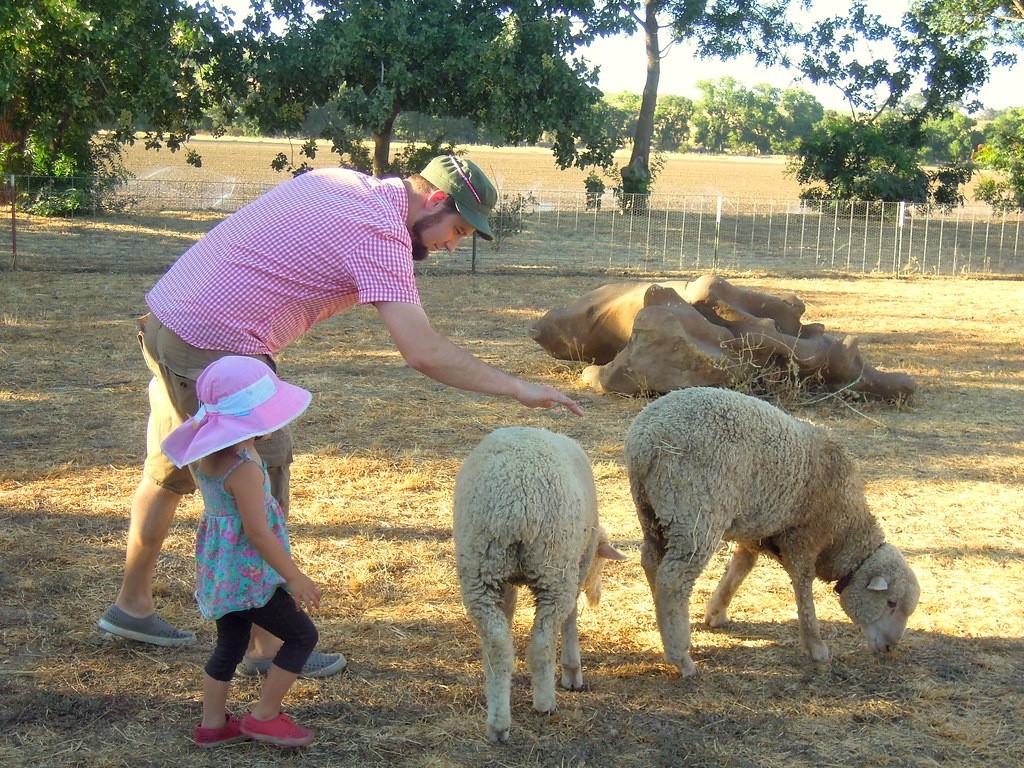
[240,711,314,747]
[98,604,198,647]
[195,712,251,748]
[234,651,347,679]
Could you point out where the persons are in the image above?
[159,356,324,747]
[99,155,588,678]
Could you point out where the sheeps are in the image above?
[453,426,629,746]
[624,386,921,677]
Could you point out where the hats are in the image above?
[420,155,498,241]
[161,356,312,469]
